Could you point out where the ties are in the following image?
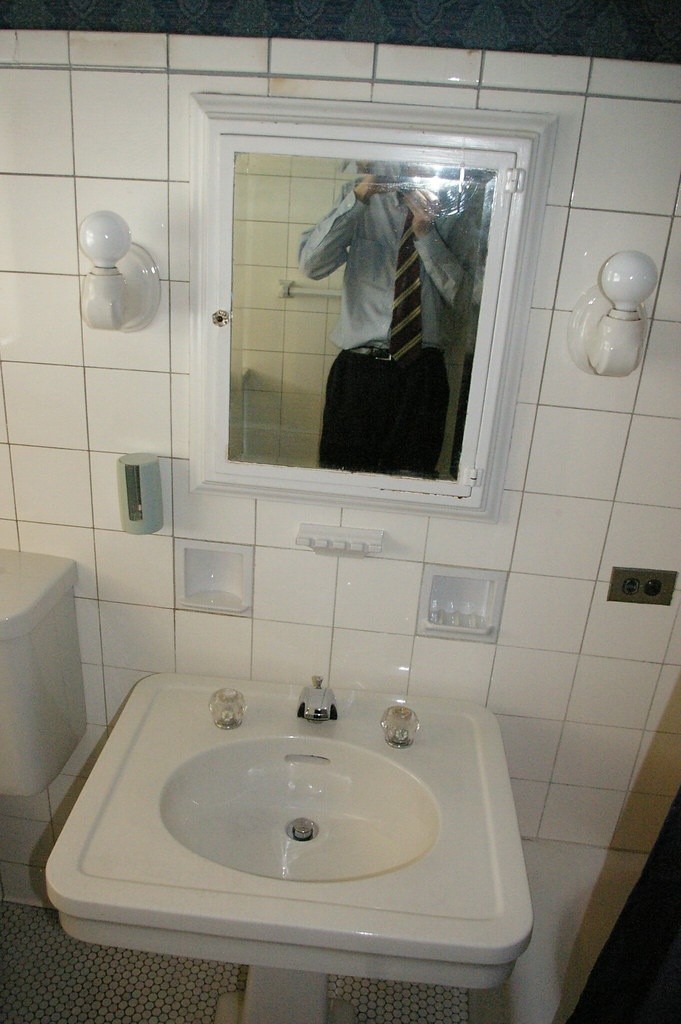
[390,207,422,370]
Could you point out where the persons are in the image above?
[297,158,494,481]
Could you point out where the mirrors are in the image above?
[224,151,501,484]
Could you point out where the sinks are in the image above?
[46,676,537,988]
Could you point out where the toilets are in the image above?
[1,548,89,800]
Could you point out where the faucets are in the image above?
[297,688,338,722]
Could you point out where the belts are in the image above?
[350,346,442,361]
